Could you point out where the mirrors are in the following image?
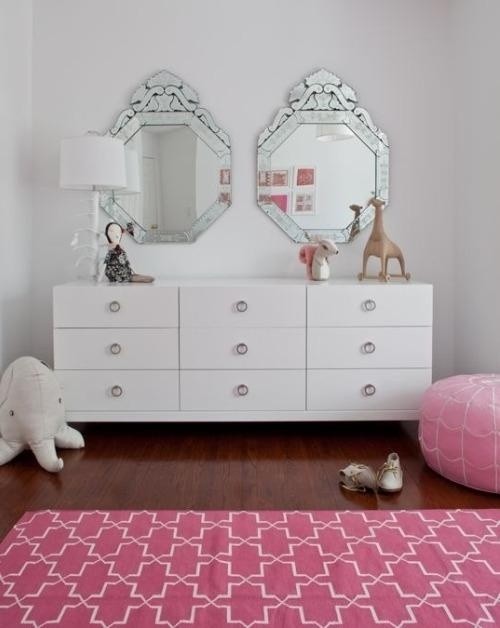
[256,68,390,243]
[100,69,233,244]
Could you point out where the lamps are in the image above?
[61,136,126,280]
[106,144,141,202]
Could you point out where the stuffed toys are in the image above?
[0,355,86,474]
[100,222,156,284]
[298,234,339,281]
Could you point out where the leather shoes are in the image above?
[340,462,377,493]
[378,453,403,492]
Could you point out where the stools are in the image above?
[416,374,500,494]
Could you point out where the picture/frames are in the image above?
[271,165,316,215]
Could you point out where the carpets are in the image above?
[0,510,500,628]
